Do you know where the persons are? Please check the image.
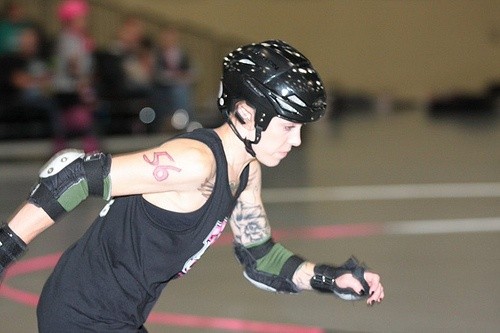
[0,0,193,157]
[0,39,385,333]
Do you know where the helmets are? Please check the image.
[218,39,327,124]
